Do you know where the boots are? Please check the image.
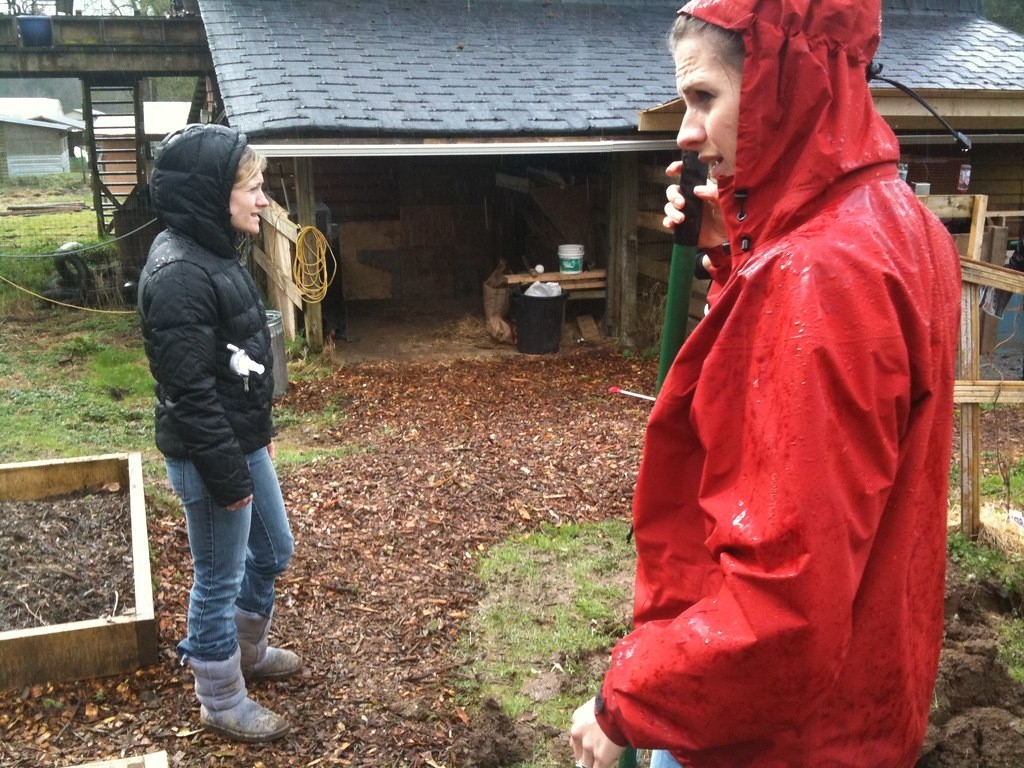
[188,641,289,741]
[234,606,303,679]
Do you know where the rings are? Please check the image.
[574,760,587,768]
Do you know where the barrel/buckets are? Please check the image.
[558,244,585,275]
[509,281,570,354]
[264,309,290,403]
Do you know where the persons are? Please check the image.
[694,248,713,280]
[135,123,302,739]
[566,0,962,768]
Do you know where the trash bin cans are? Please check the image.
[513,284,570,354]
[266,310,288,399]
